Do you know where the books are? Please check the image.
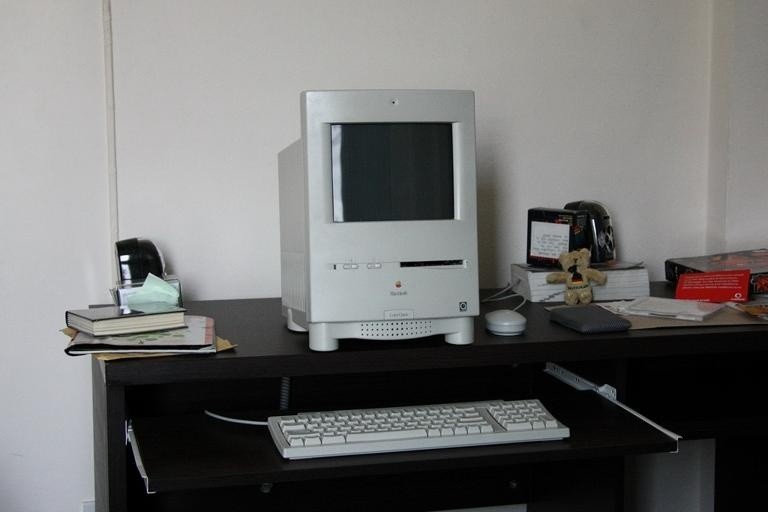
[618,295,725,324]
[508,257,651,304]
[720,293,768,321]
[63,314,218,357]
[64,303,188,338]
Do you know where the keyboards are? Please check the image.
[267,398,571,461]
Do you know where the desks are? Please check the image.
[91,297,768,511]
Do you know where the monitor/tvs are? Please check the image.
[277,88,480,352]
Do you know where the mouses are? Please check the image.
[485,309,527,336]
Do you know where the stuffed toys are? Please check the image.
[545,247,607,307]
